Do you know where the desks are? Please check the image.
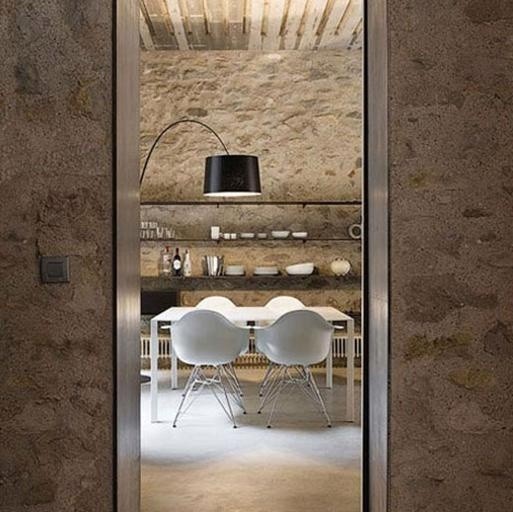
[148,306,356,424]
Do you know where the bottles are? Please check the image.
[162,246,191,277]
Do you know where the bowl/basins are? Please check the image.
[201,254,224,276]
[225,262,313,275]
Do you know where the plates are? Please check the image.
[239,231,308,240]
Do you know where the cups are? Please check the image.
[139,221,176,240]
[210,226,236,240]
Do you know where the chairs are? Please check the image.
[169,294,333,430]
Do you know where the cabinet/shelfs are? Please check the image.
[137,199,362,291]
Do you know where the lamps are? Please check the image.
[138,119,262,198]
